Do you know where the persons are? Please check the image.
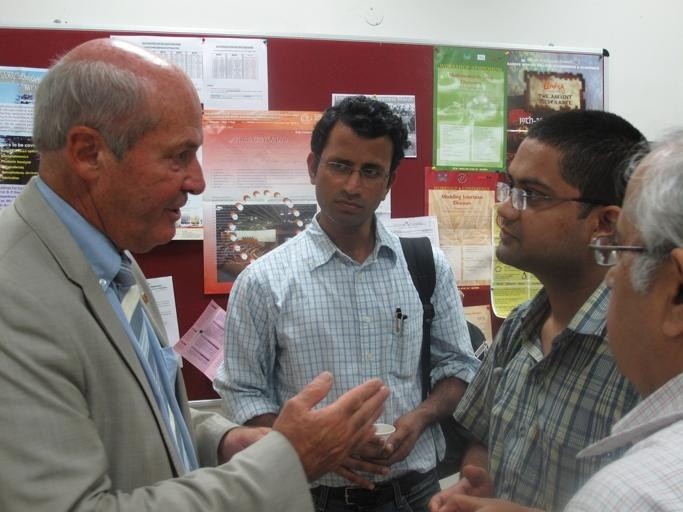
[0,38,395,512]
[422,109,652,512]
[561,127,682,512]
[209,93,482,511]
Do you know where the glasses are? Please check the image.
[591,238,649,266]
[495,181,607,212]
[314,153,393,187]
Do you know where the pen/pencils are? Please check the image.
[396,307,407,332]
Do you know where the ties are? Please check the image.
[111,255,193,474]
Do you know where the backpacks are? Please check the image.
[399,236,488,480]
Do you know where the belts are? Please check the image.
[311,466,435,508]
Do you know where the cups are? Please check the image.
[371,422,397,459]
